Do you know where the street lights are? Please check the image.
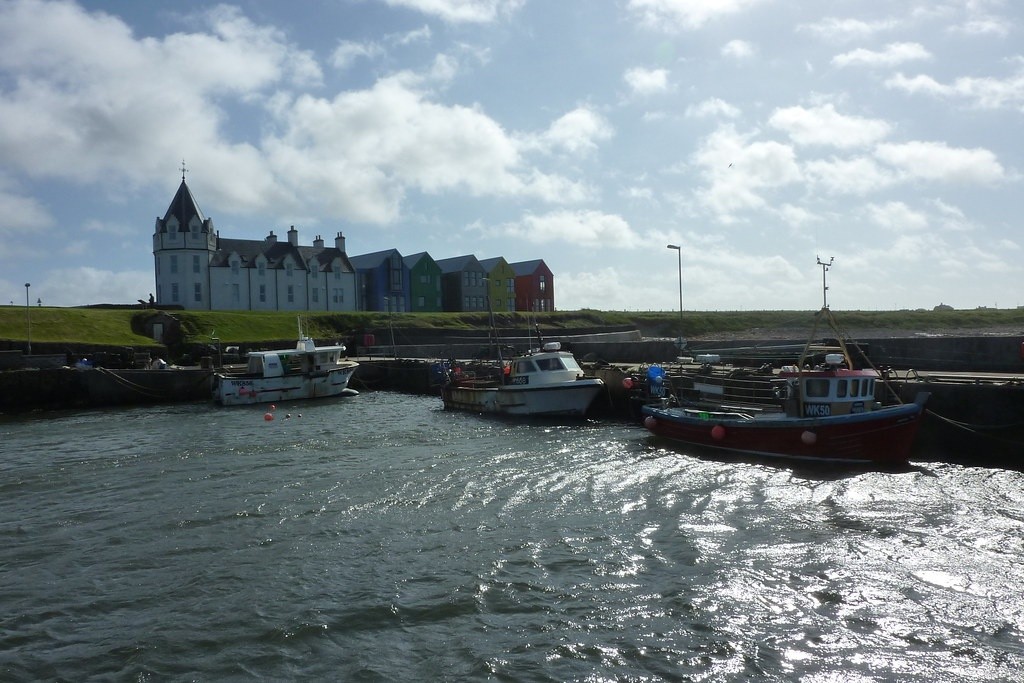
[25,282,32,354]
[667,245,682,358]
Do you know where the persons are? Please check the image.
[149,294,154,305]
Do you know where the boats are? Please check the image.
[212,314,360,408]
[430,277,607,425]
[622,254,922,464]
